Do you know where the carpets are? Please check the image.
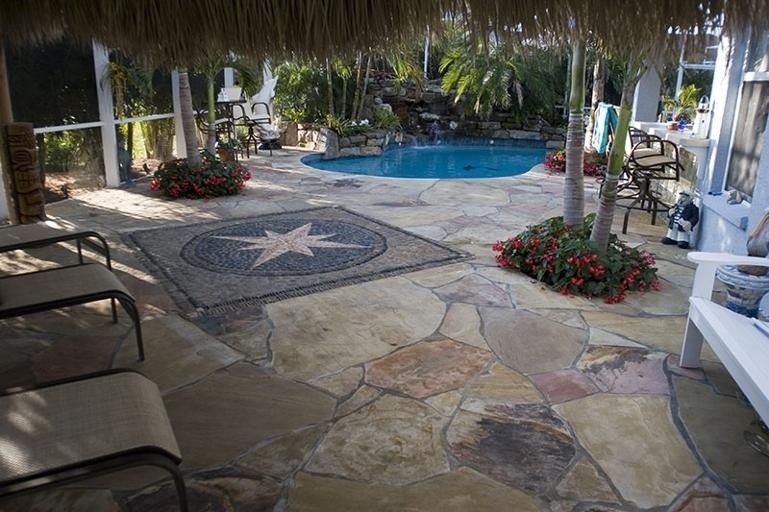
[118,203,476,320]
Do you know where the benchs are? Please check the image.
[676,252,769,431]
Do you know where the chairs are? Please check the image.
[0,219,112,271]
[192,99,275,160]
[598,101,685,236]
[1,367,191,512]
[1,262,145,362]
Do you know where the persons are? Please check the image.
[660,189,700,249]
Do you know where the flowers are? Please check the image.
[145,149,253,200]
[494,212,663,305]
[544,147,597,176]
